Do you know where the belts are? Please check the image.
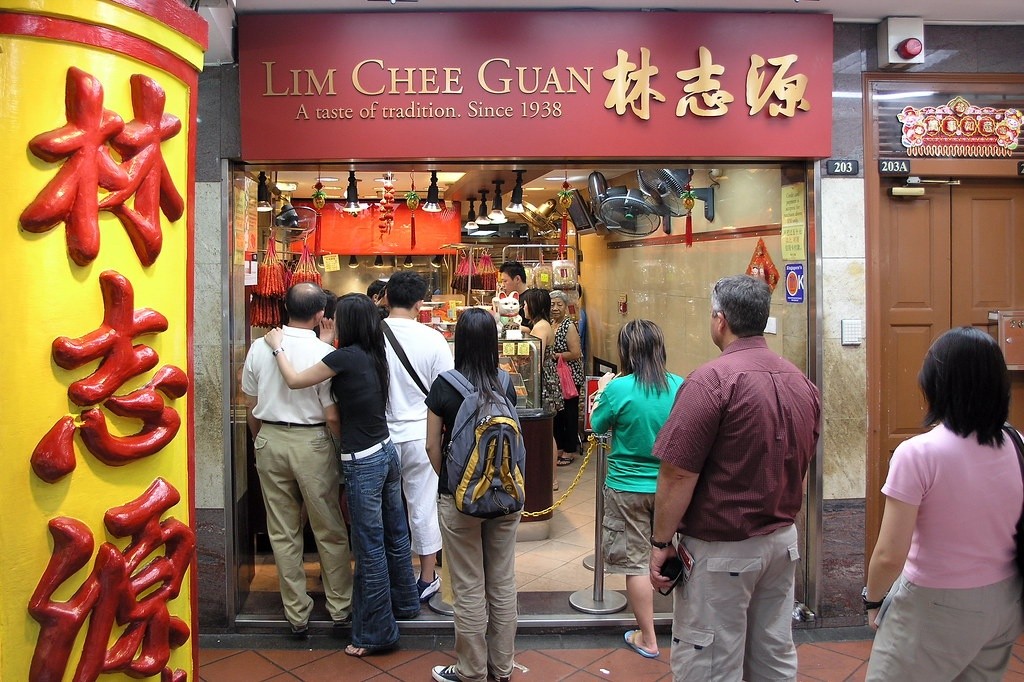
[262,419,326,427]
[340,436,391,462]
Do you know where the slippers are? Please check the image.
[557,457,574,466]
[624,629,660,658]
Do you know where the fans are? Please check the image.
[588,170,713,237]
[515,199,559,239]
[270,203,317,242]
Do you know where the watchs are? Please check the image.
[862,587,886,610]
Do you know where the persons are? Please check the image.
[263,293,420,655]
[425,308,522,682]
[590,319,684,658]
[498,261,534,331]
[649,274,820,682]
[382,271,453,601]
[523,289,566,491]
[368,281,390,318]
[241,284,354,635]
[315,290,337,338]
[861,327,1024,682]
[549,290,584,465]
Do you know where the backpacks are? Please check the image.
[438,369,527,519]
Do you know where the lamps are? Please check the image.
[319,255,442,269]
[257,170,445,212]
[465,169,524,229]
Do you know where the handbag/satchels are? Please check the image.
[556,353,580,400]
[1003,422,1024,577]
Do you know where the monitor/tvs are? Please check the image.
[565,188,596,236]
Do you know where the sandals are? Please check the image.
[345,644,375,656]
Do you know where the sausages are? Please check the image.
[251,265,323,329]
[450,270,498,293]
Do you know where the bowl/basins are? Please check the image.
[505,330,522,340]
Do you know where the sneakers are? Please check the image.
[415,568,442,604]
[489,667,510,682]
[432,665,463,682]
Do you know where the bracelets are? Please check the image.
[594,391,602,403]
[272,347,284,356]
[650,536,672,548]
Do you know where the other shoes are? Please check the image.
[332,613,352,627]
[289,621,308,635]
